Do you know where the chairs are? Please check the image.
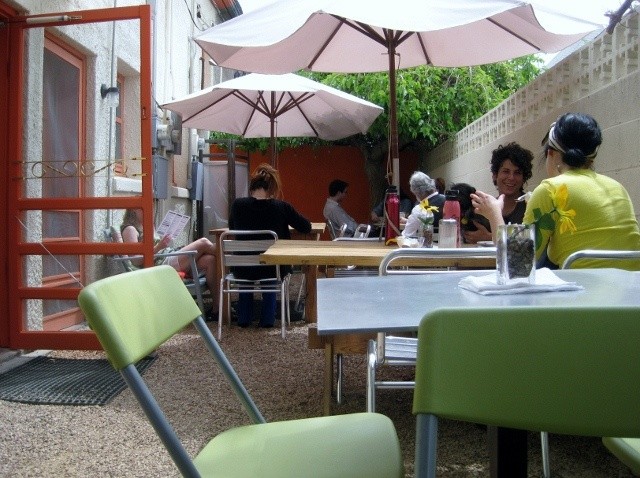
[325,219,337,239]
[72,266,402,477]
[411,306,639,478]
[365,247,501,415]
[215,230,294,341]
[106,227,213,323]
[293,224,370,321]
[332,223,347,241]
[556,251,640,272]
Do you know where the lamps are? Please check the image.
[101,84,117,99]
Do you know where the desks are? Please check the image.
[313,269,639,477]
[209,223,327,325]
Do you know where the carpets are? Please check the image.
[1,357,155,407]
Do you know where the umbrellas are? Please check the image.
[159,73,385,171]
[191,0,607,197]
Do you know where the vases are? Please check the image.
[421,225,435,247]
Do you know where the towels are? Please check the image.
[458,267,583,296]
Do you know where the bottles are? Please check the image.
[438,219,457,248]
[385,185,400,245]
[443,190,461,247]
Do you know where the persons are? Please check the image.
[370,189,412,222]
[463,143,533,243]
[323,179,359,238]
[402,171,445,243]
[120,208,217,314]
[228,164,311,329]
[469,113,640,272]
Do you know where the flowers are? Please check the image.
[417,199,439,229]
[512,183,577,249]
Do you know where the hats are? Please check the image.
[541,123,599,158]
